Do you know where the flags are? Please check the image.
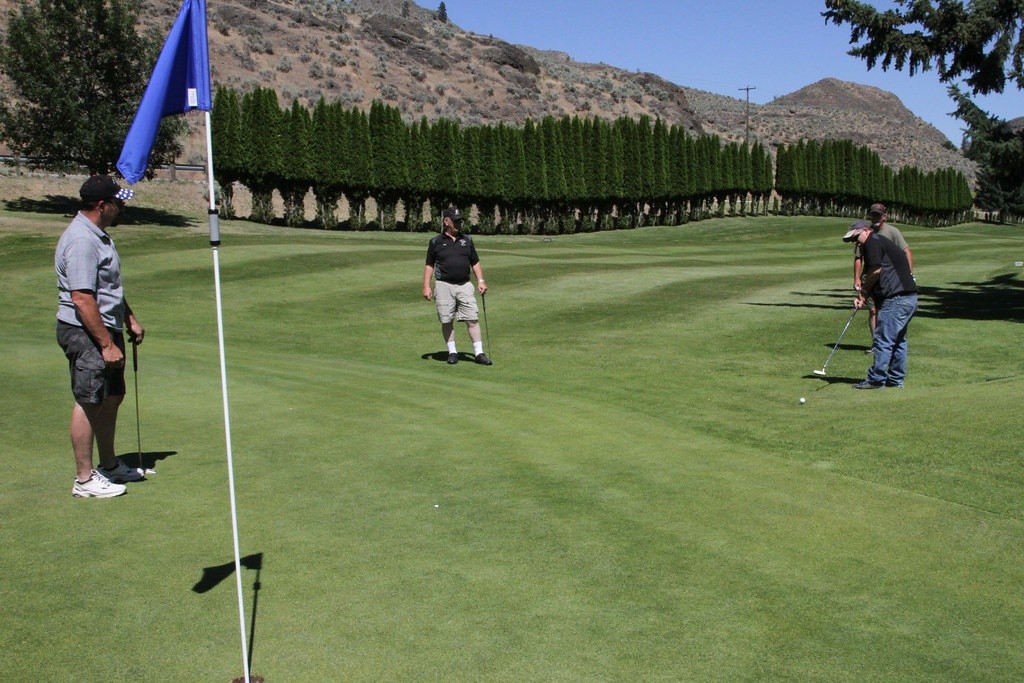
[117,0,214,184]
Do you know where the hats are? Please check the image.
[842,220,868,243]
[870,204,886,214]
[444,207,466,221]
[80,174,134,202]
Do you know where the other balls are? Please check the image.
[800,398,805,403]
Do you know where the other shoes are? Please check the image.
[865,347,876,353]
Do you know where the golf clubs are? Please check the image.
[482,293,491,360]
[813,305,860,376]
[132,338,157,474]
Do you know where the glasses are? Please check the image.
[448,217,463,223]
[104,200,126,208]
[850,234,859,241]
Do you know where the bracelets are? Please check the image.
[478,279,486,283]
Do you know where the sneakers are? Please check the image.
[884,382,904,389]
[71,468,127,499]
[475,353,492,365]
[853,381,883,389]
[447,353,457,364]
[95,456,144,482]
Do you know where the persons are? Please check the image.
[422,207,493,366]
[54,174,145,497]
[854,204,912,354]
[843,222,918,389]
